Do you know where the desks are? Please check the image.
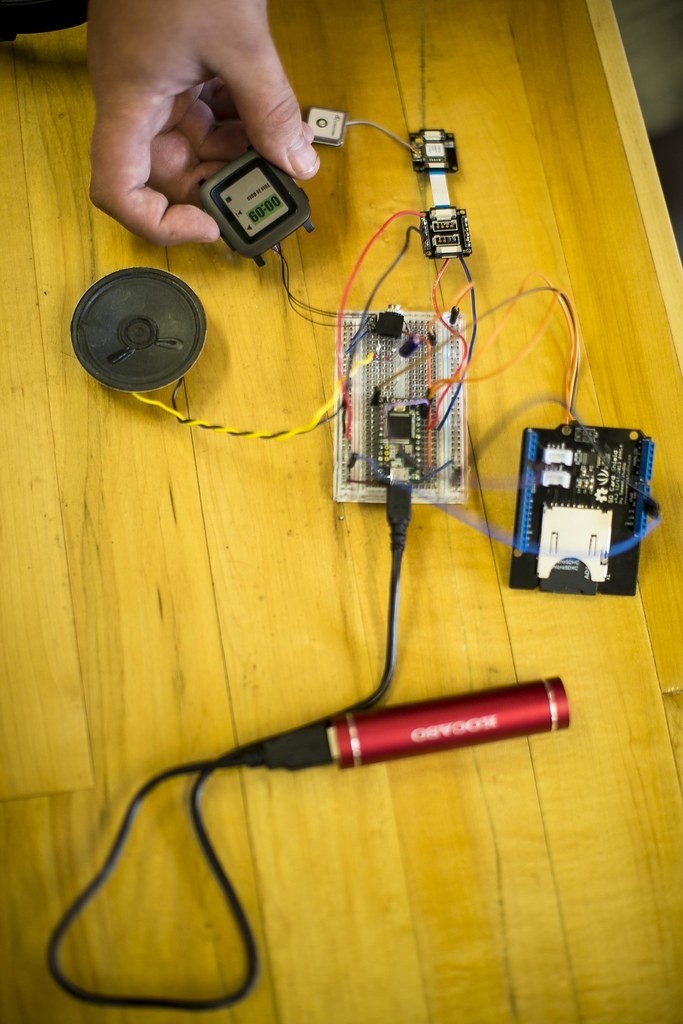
[0,3,682,1024]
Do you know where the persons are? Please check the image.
[0,0,320,247]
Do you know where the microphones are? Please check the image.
[238,683,570,774]
